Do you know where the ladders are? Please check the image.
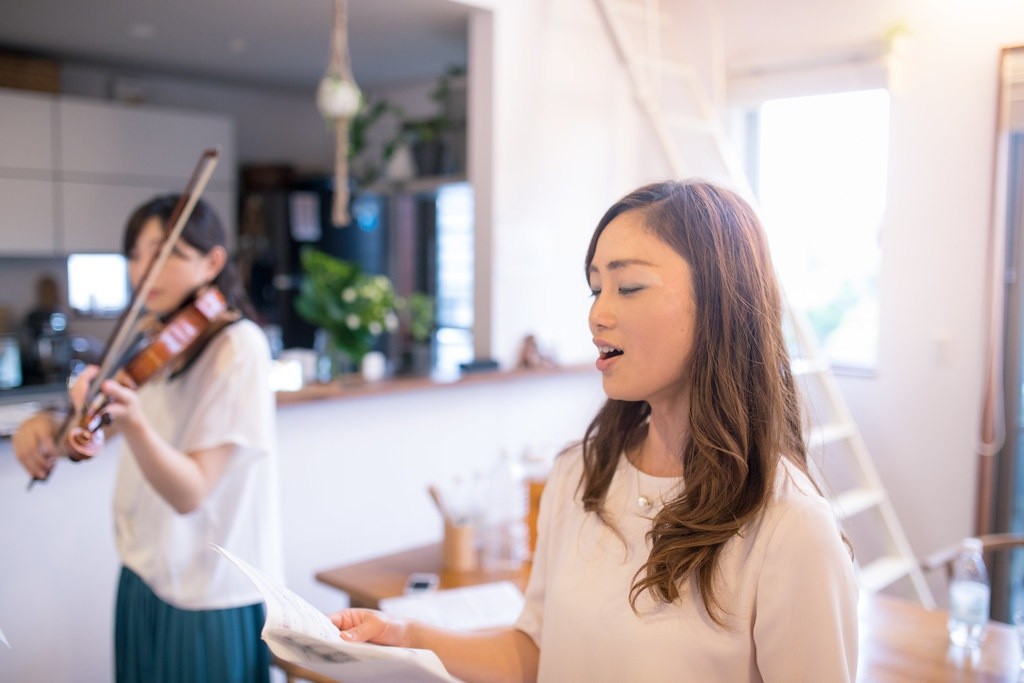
[593,0,935,613]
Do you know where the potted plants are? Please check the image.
[299,250,433,392]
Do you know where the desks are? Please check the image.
[315,517,1024,683]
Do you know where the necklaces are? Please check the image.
[636,429,685,511]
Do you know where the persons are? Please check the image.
[323,174,866,683]
[21,276,97,386]
[9,195,287,683]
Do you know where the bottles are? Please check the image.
[948,538,991,649]
[482,449,530,569]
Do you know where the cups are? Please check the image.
[444,519,475,571]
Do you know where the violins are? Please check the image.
[61,284,229,463]
